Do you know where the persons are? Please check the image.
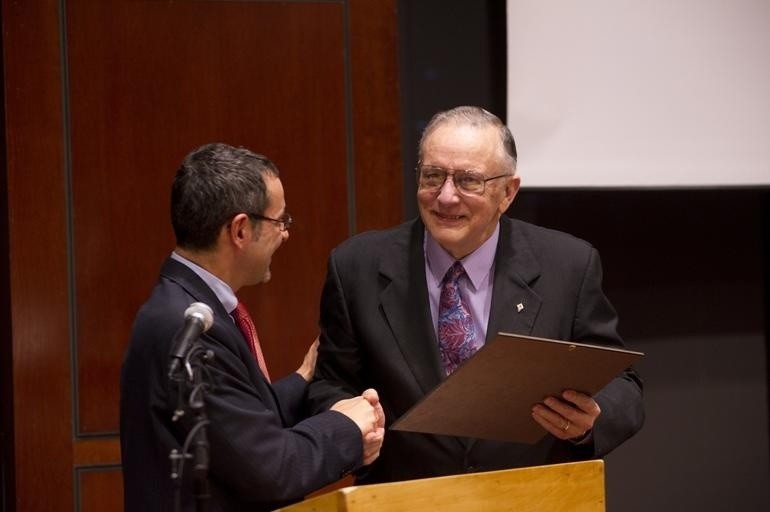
[301,106,645,483]
[120,141,379,512]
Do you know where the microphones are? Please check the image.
[165,301,214,380]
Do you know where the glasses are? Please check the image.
[415,163,514,199]
[226,210,292,232]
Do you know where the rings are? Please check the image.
[563,420,570,433]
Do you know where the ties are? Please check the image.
[229,302,272,387]
[437,263,478,377]
